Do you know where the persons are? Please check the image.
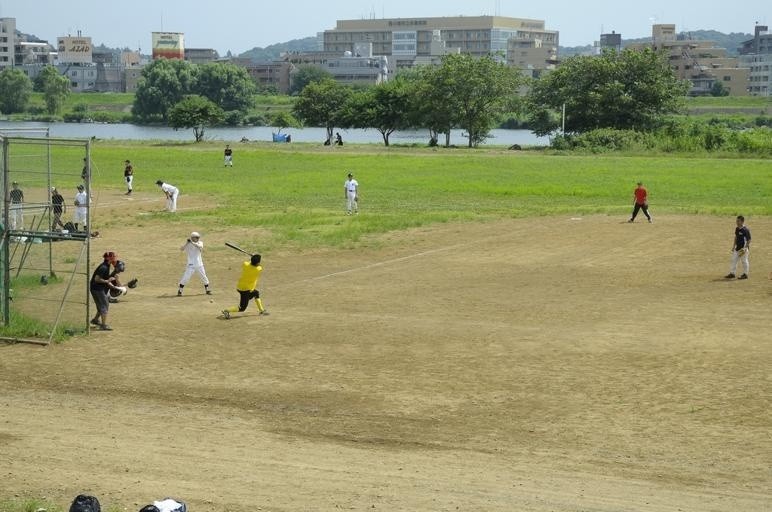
[80,158,92,188]
[331,131,344,146]
[177,231,213,296]
[224,143,234,167]
[73,185,89,229]
[90,252,127,332]
[156,180,179,210]
[345,171,359,215]
[629,182,655,223]
[123,159,133,195]
[222,254,270,320]
[726,214,752,283]
[48,185,68,227]
[69,494,101,512]
[8,181,26,227]
[139,504,160,512]
[107,262,137,304]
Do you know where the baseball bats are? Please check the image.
[225,243,253,255]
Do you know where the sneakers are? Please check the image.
[725,272,751,282]
[345,210,359,217]
[205,290,213,296]
[161,207,177,213]
[628,217,655,225]
[257,309,271,317]
[124,192,133,196]
[90,317,113,332]
[220,308,230,321]
[177,289,184,297]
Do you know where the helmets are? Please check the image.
[101,251,118,262]
[114,259,125,273]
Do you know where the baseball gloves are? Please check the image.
[128,279,138,288]
[737,247,748,258]
[229,157,232,161]
[127,177,130,182]
[354,195,359,202]
[642,201,648,209]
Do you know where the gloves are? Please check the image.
[126,177,131,183]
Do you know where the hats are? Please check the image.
[155,179,164,185]
[124,159,131,164]
[11,180,20,186]
[50,186,56,194]
[190,231,200,239]
[347,172,353,177]
[76,184,85,192]
